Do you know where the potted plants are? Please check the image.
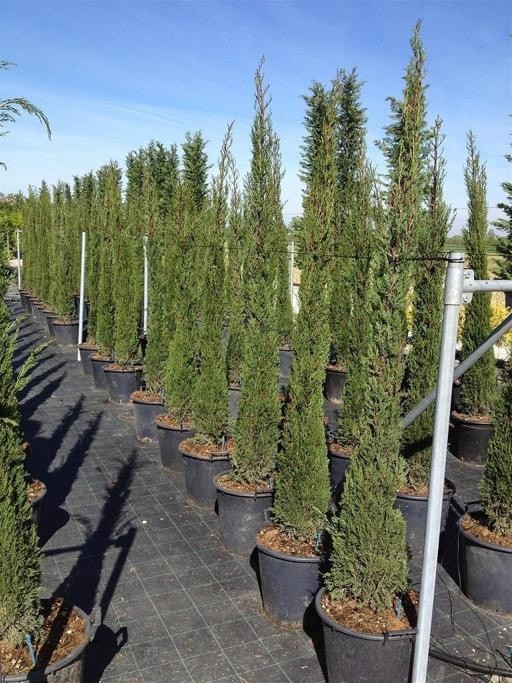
[0,18,512,683]
